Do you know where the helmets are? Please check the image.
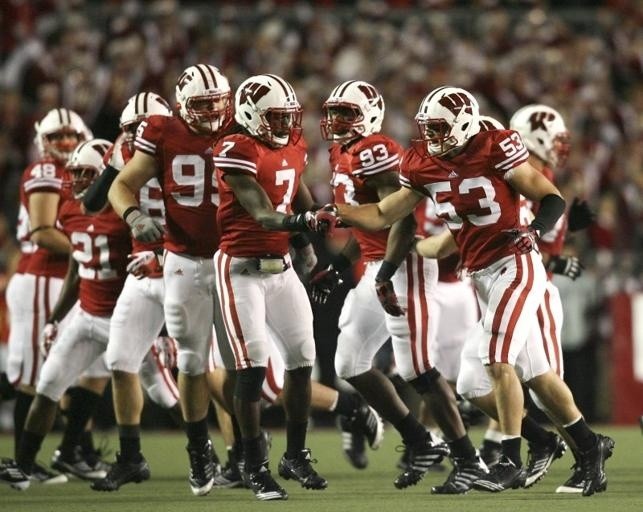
[69,135,116,196]
[412,85,480,157]
[510,103,569,168]
[320,80,386,146]
[36,107,93,162]
[174,63,232,132]
[119,92,174,156]
[481,113,507,131]
[234,72,304,147]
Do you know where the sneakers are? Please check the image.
[471,454,523,490]
[243,462,288,501]
[555,458,585,493]
[479,441,500,462]
[50,443,107,481]
[522,431,567,488]
[278,448,328,489]
[0,456,31,491]
[19,461,68,484]
[186,439,214,497]
[350,394,384,451]
[215,452,246,488]
[580,432,615,497]
[430,456,490,494]
[90,452,151,492]
[393,432,450,489]
[336,413,368,469]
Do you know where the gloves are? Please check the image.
[309,252,352,304]
[126,250,162,280]
[314,202,340,239]
[121,206,166,244]
[568,197,594,231]
[282,209,315,235]
[151,326,176,370]
[40,317,58,361]
[545,253,584,281]
[501,222,544,256]
[374,260,406,318]
